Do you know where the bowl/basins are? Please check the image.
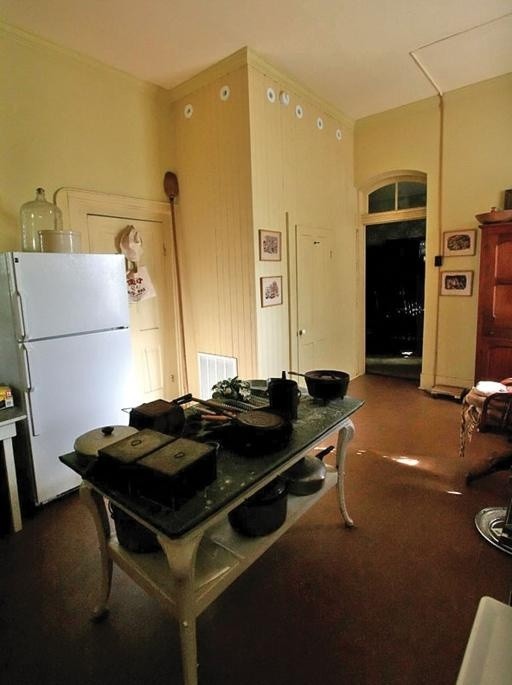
[304,370,350,405]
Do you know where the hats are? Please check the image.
[119,226,143,262]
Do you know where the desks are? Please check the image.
[56,383,367,685]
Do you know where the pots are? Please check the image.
[227,476,288,538]
[37,230,81,254]
[198,406,293,449]
[276,445,335,497]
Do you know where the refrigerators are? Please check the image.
[0,250,143,507]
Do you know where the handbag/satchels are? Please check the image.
[466,380,507,407]
[127,265,156,303]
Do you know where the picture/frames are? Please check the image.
[258,228,283,309]
[438,227,477,297]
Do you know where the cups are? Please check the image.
[268,380,299,420]
